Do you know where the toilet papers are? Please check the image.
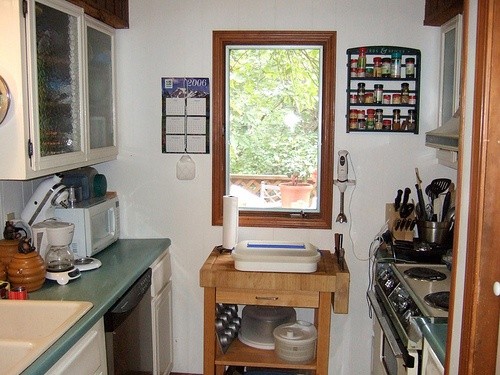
[222,194,239,250]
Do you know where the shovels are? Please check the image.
[430,178,451,199]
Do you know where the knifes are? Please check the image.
[393,188,417,233]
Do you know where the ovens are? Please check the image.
[367,264,426,375]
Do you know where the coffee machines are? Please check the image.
[32,221,82,286]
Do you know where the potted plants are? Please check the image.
[279,158,314,209]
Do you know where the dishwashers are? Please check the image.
[98,267,157,374]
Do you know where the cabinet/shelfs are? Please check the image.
[436,13,464,172]
[345,45,422,135]
[0,0,119,182]
[113,246,174,375]
[198,244,352,375]
[43,315,109,375]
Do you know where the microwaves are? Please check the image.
[43,190,122,262]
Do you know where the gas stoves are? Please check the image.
[388,262,453,322]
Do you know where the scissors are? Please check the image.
[399,203,414,218]
